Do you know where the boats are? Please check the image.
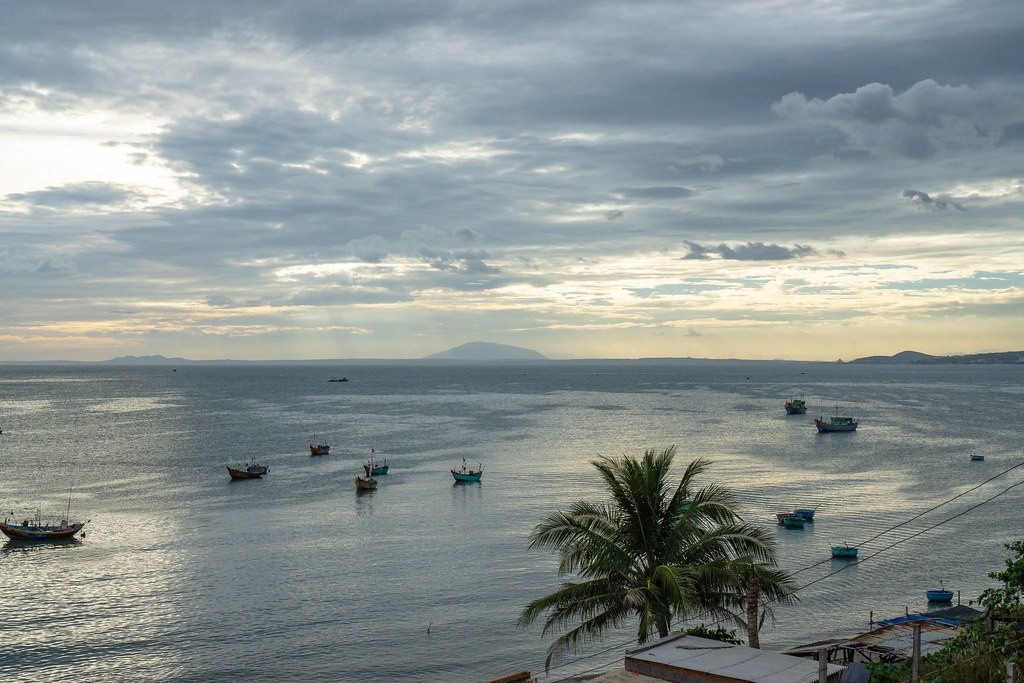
[225,465,261,479]
[794,509,814,519]
[831,546,858,557]
[308,445,330,455]
[355,476,378,489]
[969,453,986,462]
[813,418,858,432]
[363,463,389,476]
[775,512,794,522]
[247,465,270,474]
[784,517,805,526]
[925,589,954,601]
[327,377,350,384]
[450,469,483,481]
[782,399,808,414]
[0,510,97,540]
